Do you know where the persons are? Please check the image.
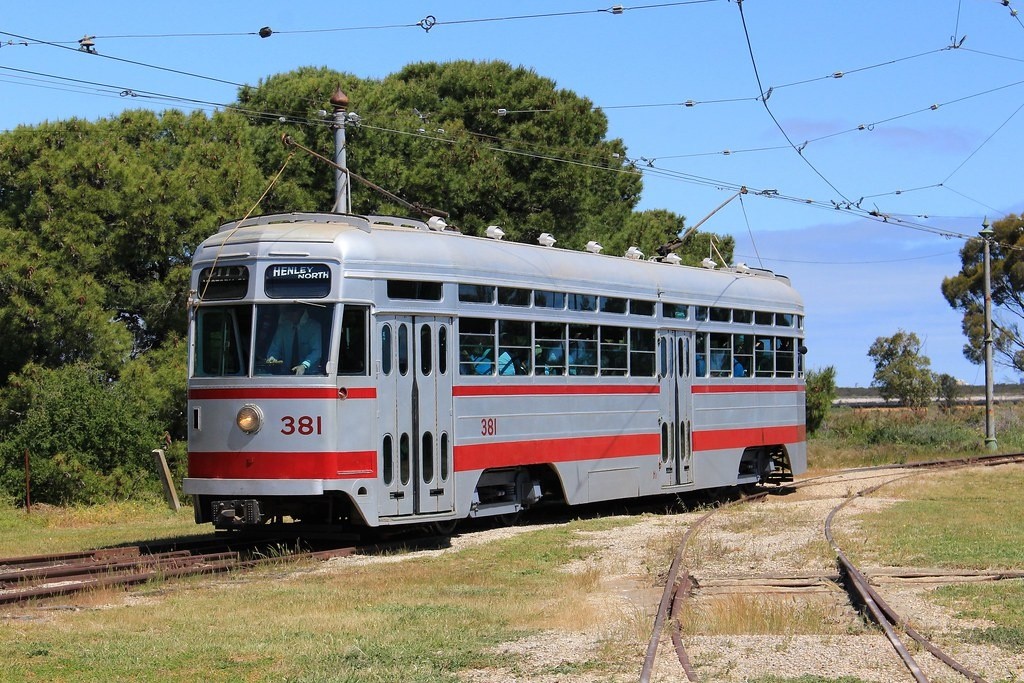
[265,304,323,375]
[460,327,746,377]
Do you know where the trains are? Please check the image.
[153,129,808,544]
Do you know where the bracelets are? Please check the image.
[302,364,308,370]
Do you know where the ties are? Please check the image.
[291,325,300,370]
